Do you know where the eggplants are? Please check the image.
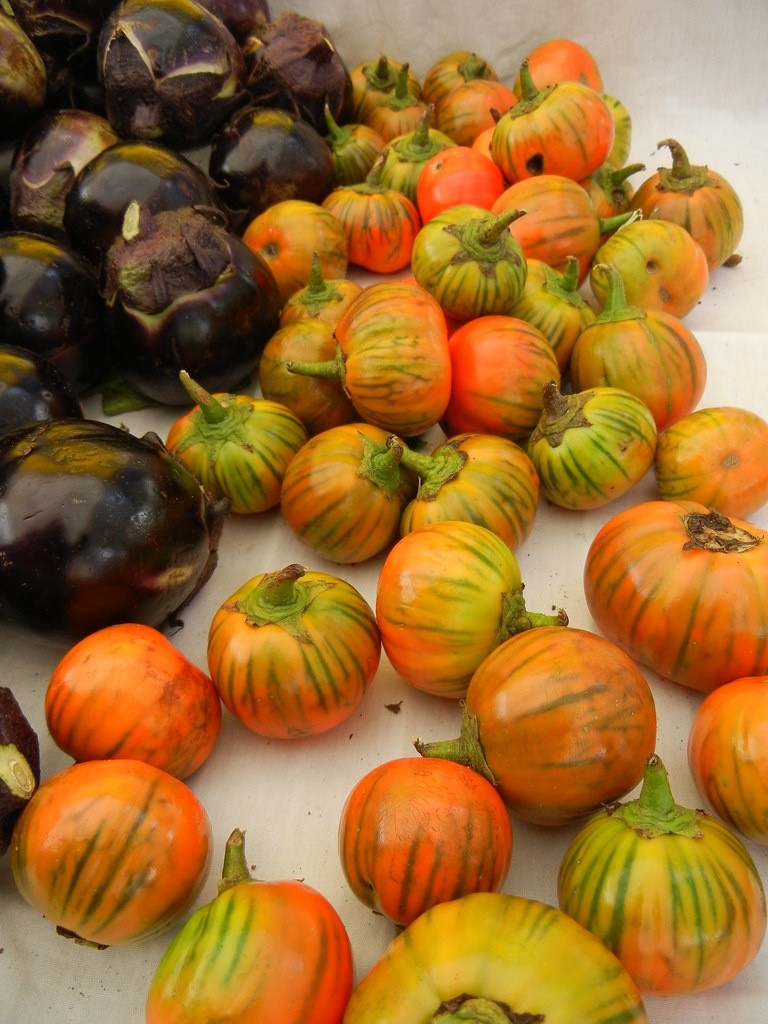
[0,0,357,828]
